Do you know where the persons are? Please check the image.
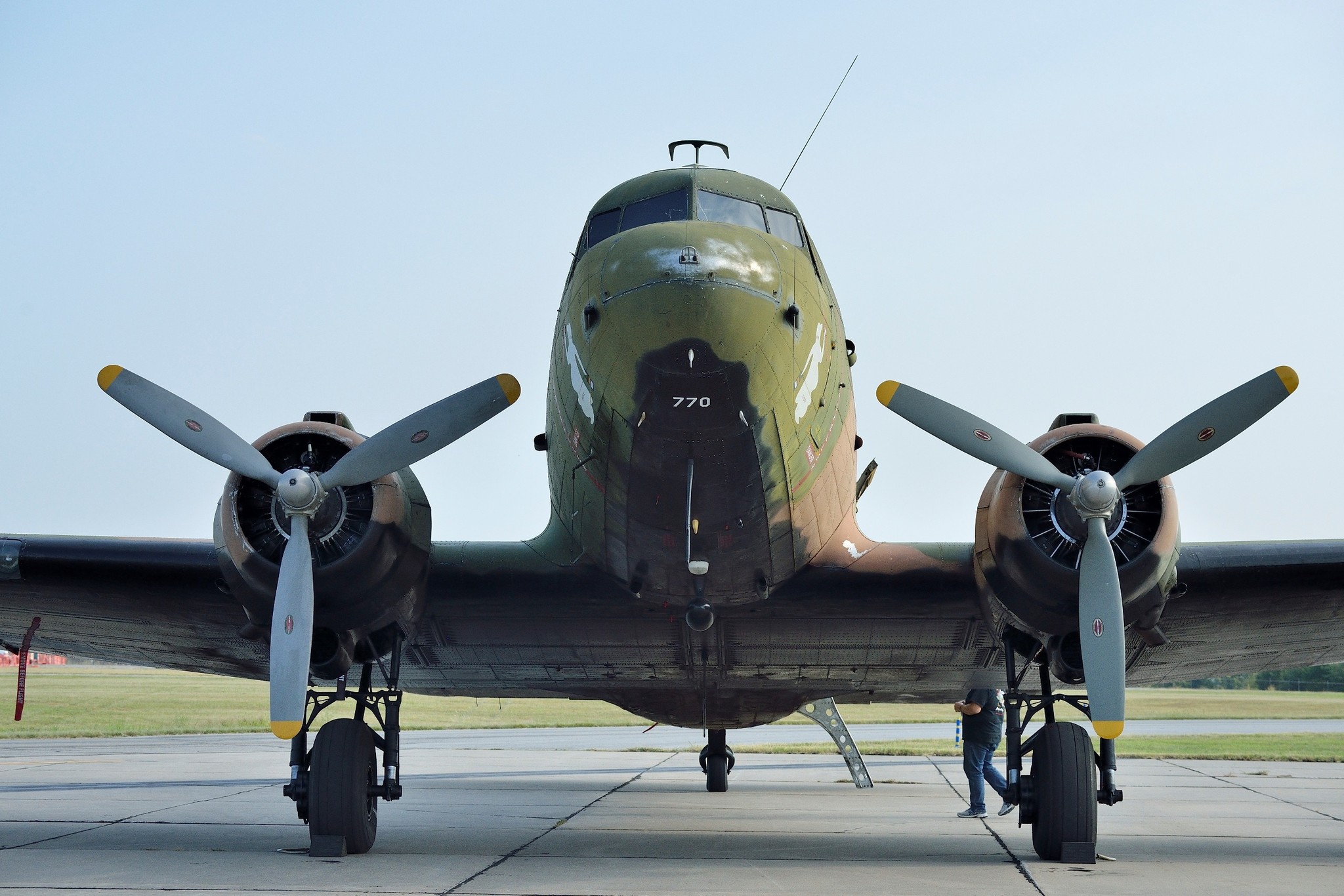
[953,688,1015,818]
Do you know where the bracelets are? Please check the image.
[960,704,964,711]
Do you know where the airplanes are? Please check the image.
[0,135,1343,866]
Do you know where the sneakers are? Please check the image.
[998,801,1015,816]
[957,808,988,818]
